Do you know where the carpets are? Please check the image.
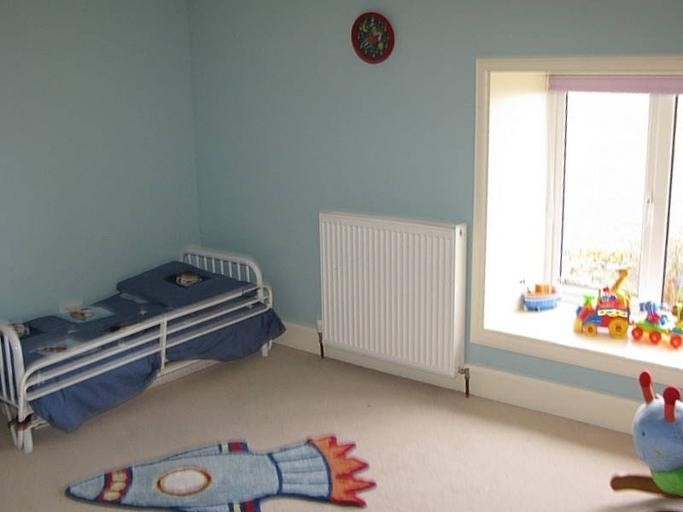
[63,433,376,512]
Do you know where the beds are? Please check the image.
[1,248,275,456]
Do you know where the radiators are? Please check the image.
[317,211,468,379]
